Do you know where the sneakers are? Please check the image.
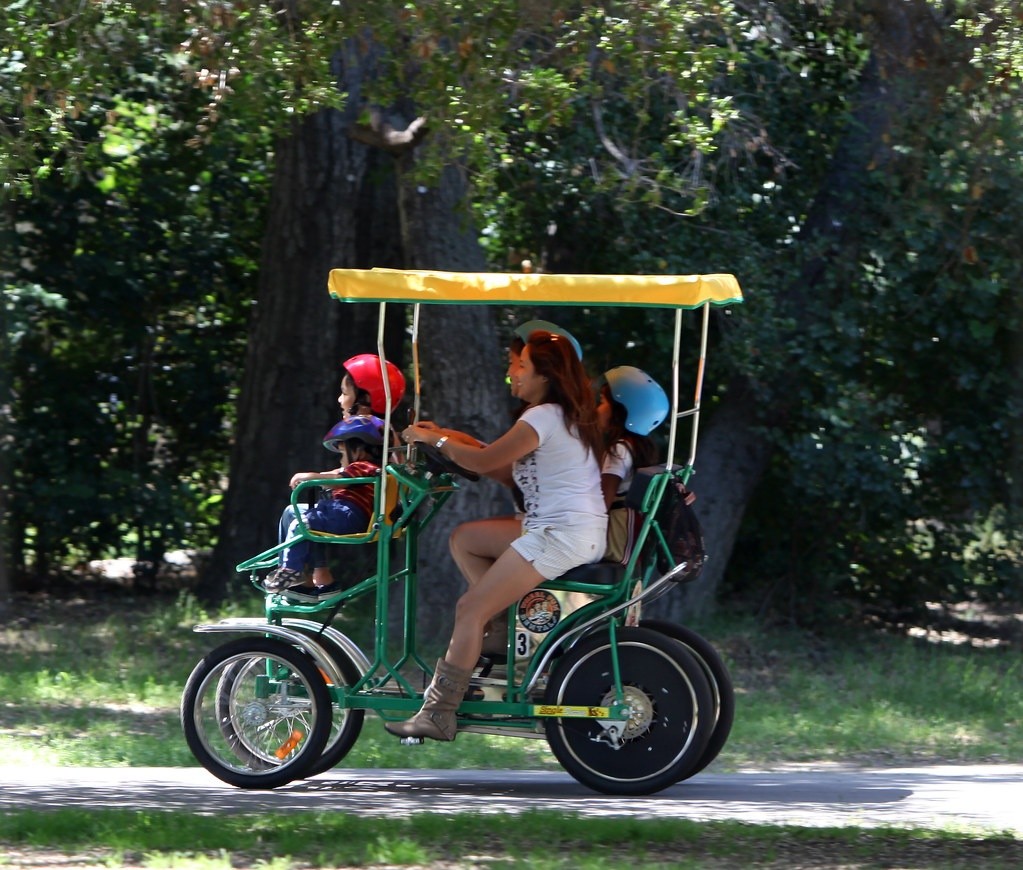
[261,568,308,592]
[278,580,343,603]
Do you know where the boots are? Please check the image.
[442,608,509,665]
[384,657,472,741]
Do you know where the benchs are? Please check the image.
[290,463,463,545]
[505,463,695,705]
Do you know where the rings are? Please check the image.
[405,436,409,439]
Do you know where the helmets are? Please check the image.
[513,320,582,362]
[343,354,406,414]
[604,366,669,436]
[322,414,394,454]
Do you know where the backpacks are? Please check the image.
[657,478,705,582]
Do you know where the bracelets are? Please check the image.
[435,436,448,449]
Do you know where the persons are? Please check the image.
[505,319,670,567]
[382,327,623,743]
[265,415,394,593]
[277,352,407,603]
